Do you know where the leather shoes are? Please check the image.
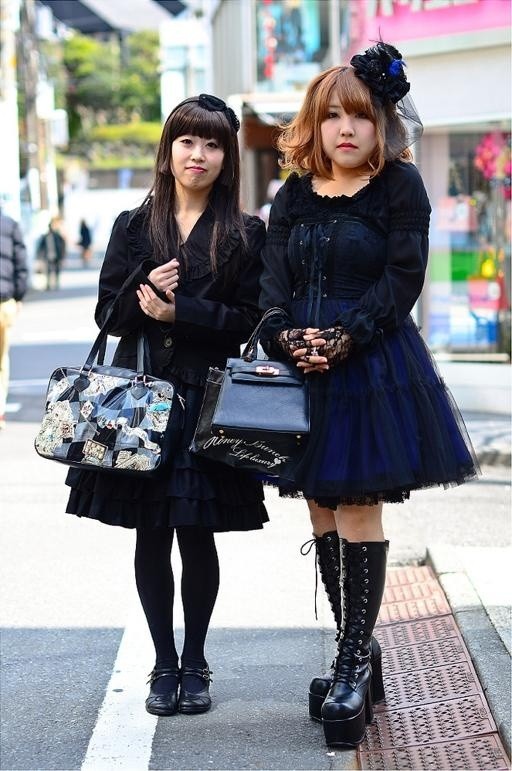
[145,668,180,715]
[178,662,211,712]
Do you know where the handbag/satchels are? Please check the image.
[34,362,187,471]
[190,357,323,483]
[212,357,313,443]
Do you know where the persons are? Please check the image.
[37,222,65,292]
[257,40,480,751]
[65,95,266,712]
[0,204,30,430]
[77,219,91,270]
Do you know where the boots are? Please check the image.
[309,530,384,721]
[320,538,389,746]
